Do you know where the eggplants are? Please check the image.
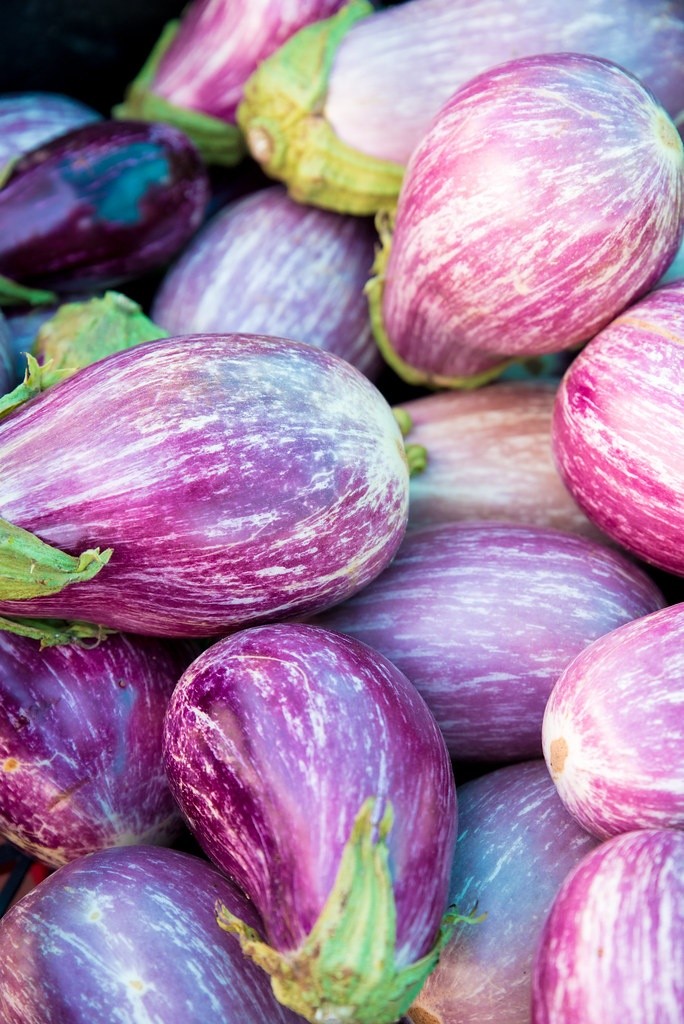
[1,0,684,1024]
